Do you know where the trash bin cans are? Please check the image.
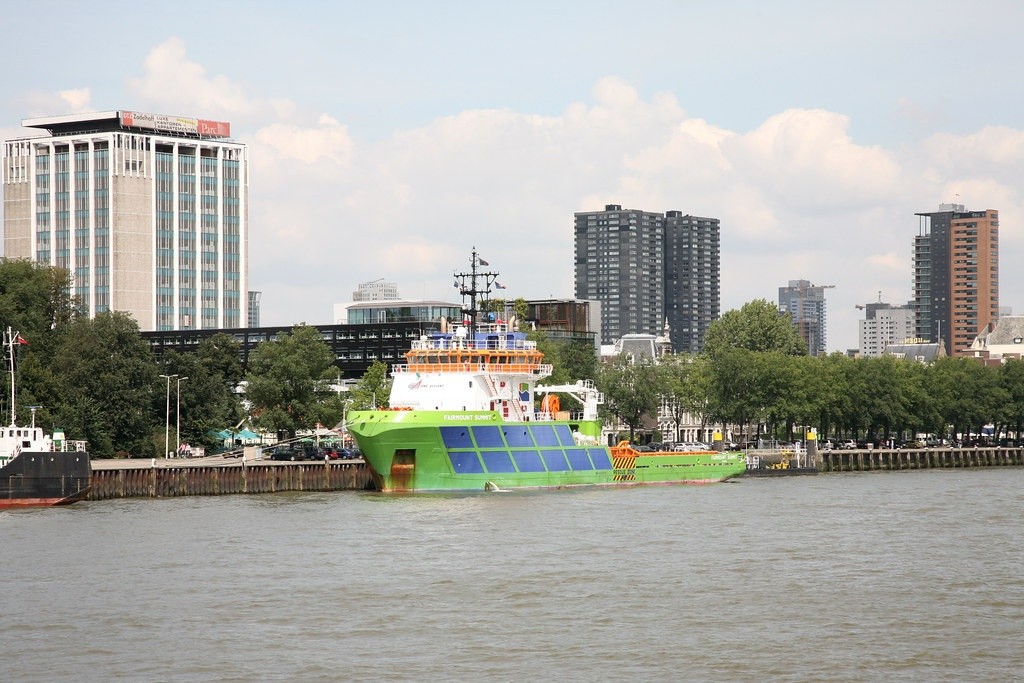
[169,450,174,459]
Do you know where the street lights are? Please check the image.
[176,377,188,459]
[158,373,178,460]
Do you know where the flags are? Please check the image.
[453,281,458,287]
[480,259,489,265]
[17,335,31,346]
[495,282,505,289]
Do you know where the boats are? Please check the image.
[342,244,748,494]
[0,323,93,510]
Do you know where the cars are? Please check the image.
[270,441,364,461]
[629,435,782,453]
[819,431,953,449]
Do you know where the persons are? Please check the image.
[879,436,891,449]
[654,444,674,451]
[958,439,963,448]
[333,443,337,449]
[843,441,847,449]
[932,435,936,440]
[179,442,191,458]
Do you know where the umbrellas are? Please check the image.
[298,437,343,445]
[217,428,259,447]
